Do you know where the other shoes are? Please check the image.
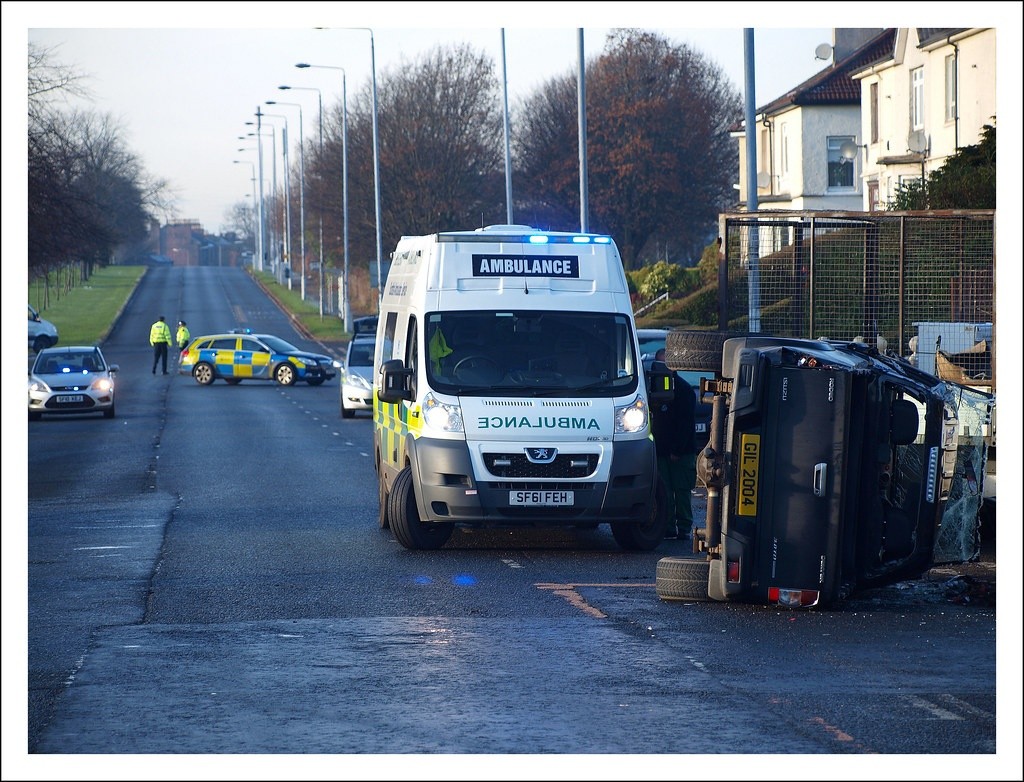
[664,531,677,537]
[163,371,169,375]
[677,530,690,540]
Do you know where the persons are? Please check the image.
[150,316,172,375]
[177,321,190,353]
[656,348,697,540]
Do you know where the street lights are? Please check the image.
[233,112,293,288]
[294,62,351,332]
[278,85,327,319]
[266,100,306,302]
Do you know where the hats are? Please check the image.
[178,321,186,325]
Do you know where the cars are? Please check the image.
[635,327,715,446]
[28,345,120,419]
[338,315,395,418]
[176,326,337,388]
[28,304,59,353]
[652,327,995,613]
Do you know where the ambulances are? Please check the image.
[370,223,675,554]
